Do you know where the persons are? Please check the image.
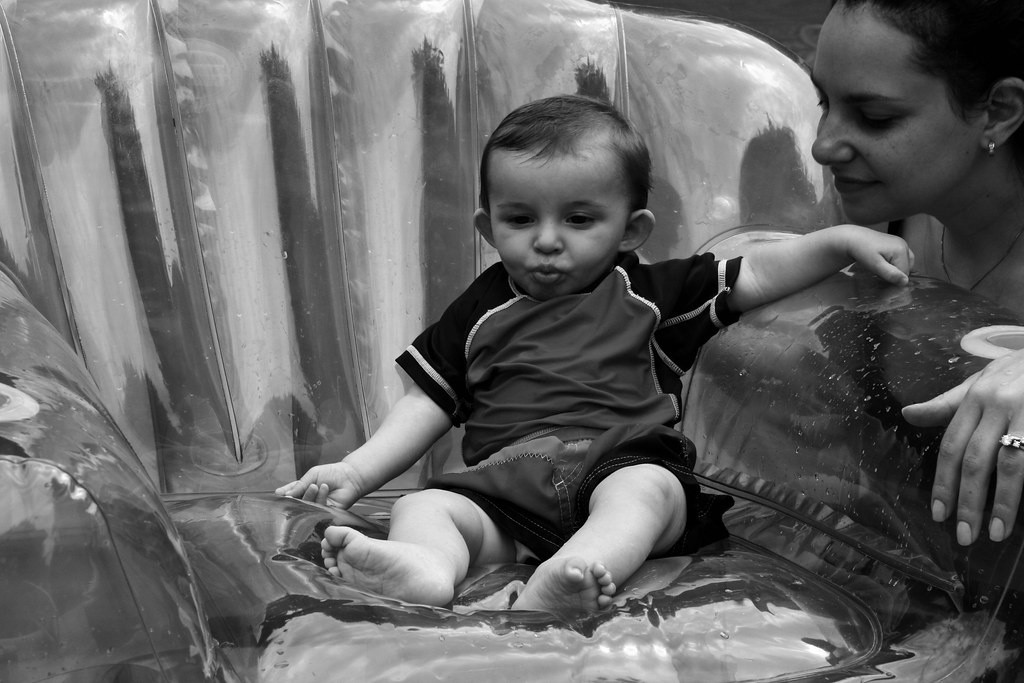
[810,1,1024,545]
[276,95,915,612]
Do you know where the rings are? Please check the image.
[999,434,1024,452]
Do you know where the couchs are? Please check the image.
[0,0,1024,683]
